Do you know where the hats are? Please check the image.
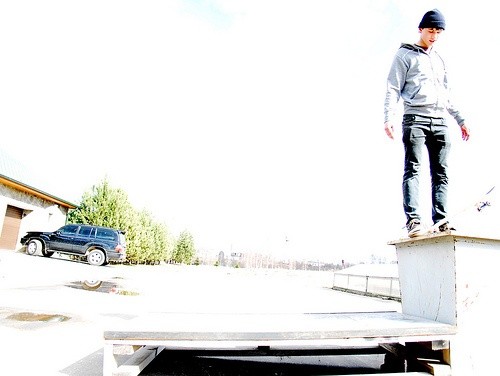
[418,8,446,30]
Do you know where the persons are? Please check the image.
[384,8,471,238]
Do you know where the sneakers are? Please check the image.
[402,217,427,237]
[431,219,458,230]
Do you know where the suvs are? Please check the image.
[20,223,128,267]
